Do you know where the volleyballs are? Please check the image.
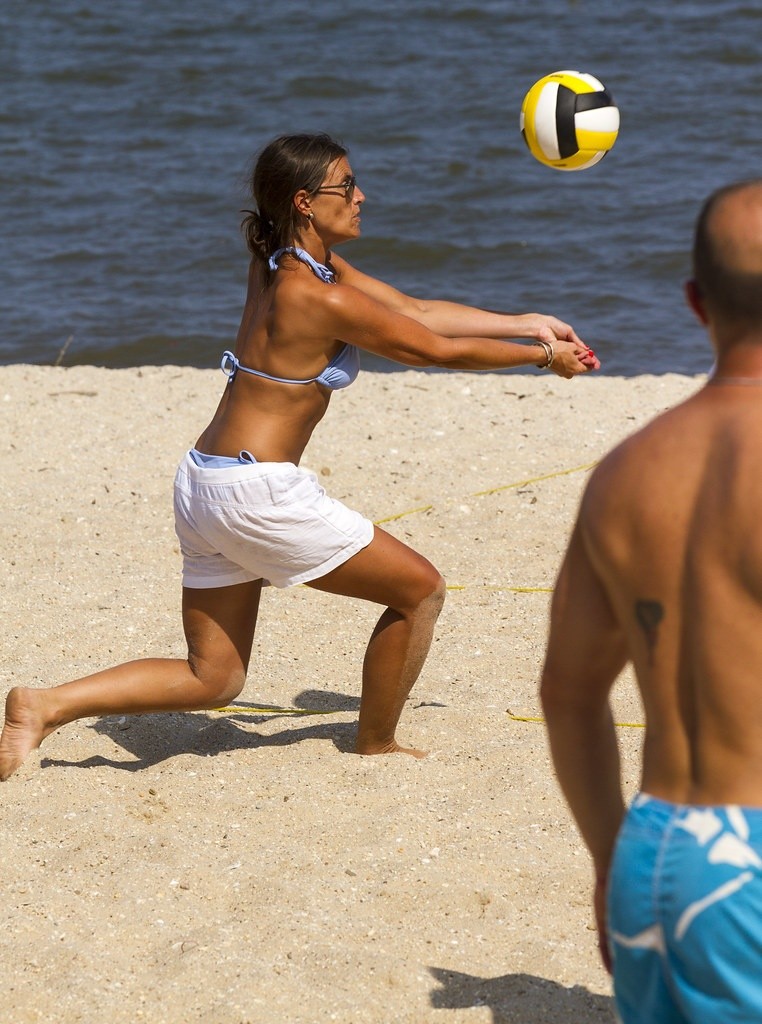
[519,69,621,173]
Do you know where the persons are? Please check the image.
[539,180,762,1024]
[0,134,601,780]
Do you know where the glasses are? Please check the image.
[317,176,355,202]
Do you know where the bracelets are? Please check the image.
[532,341,554,371]
